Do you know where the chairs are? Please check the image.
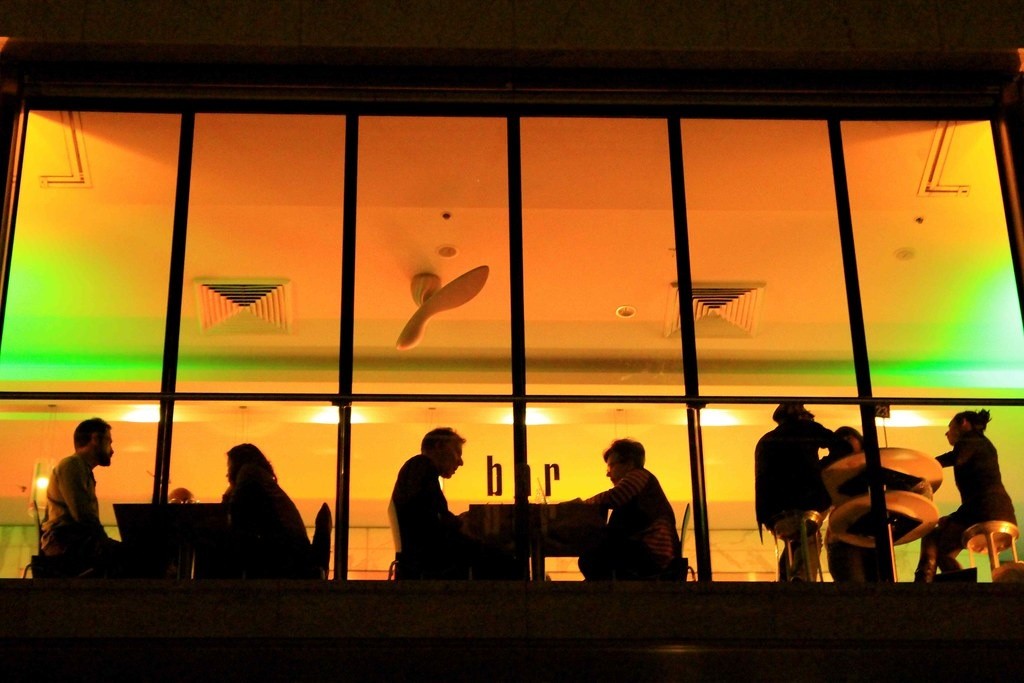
[313,503,334,586]
[25,498,117,580]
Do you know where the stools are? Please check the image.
[773,512,828,583]
[964,519,1020,584]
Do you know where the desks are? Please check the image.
[115,503,234,594]
[470,503,607,580]
[823,447,944,578]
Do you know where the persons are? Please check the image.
[392,428,517,581]
[40,419,139,579]
[755,402,1016,583]
[221,444,319,579]
[578,440,681,581]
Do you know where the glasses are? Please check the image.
[607,464,617,474]
